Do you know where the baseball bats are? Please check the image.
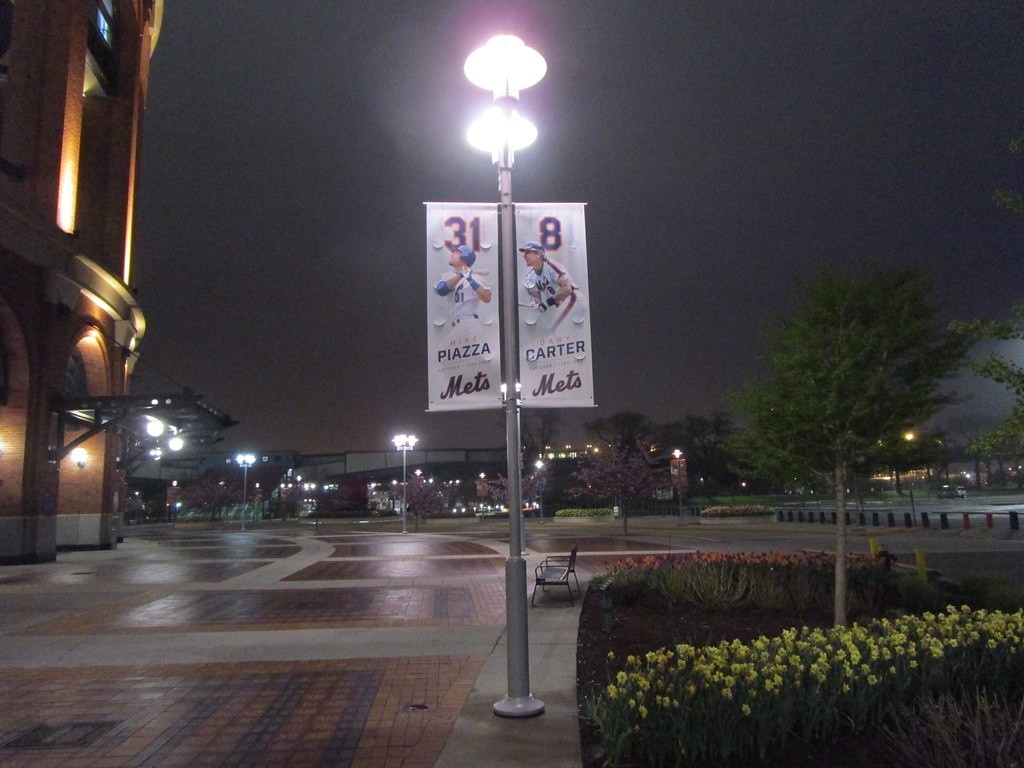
[473,269,490,276]
[518,302,534,308]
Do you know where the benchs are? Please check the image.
[530,544,579,608]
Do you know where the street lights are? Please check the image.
[461,32,550,722]
[235,455,256,533]
[534,460,546,525]
[391,434,419,535]
[671,449,688,526]
[280,483,293,521]
[171,480,178,529]
[478,472,486,522]
[296,476,302,527]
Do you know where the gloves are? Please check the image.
[535,301,550,313]
[458,268,475,281]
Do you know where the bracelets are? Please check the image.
[471,283,480,290]
[546,297,555,306]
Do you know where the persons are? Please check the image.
[518,242,587,338]
[436,245,490,337]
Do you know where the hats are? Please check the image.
[518,242,545,255]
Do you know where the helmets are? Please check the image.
[451,245,476,266]
